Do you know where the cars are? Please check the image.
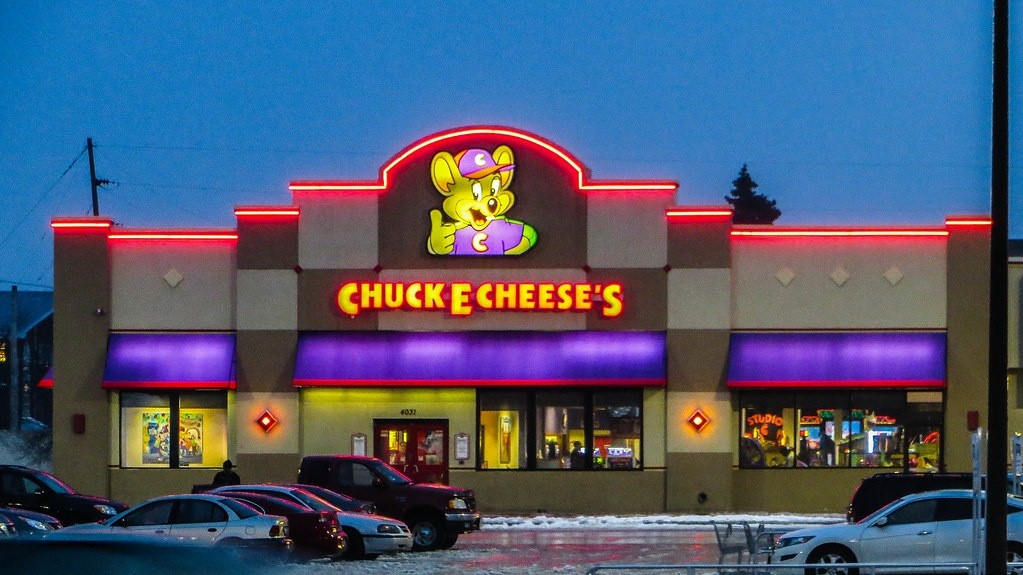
[768,488,1023,575]
[0,507,63,537]
[264,482,378,516]
[49,493,292,575]
[202,485,414,562]
[205,491,346,561]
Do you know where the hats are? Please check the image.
[223,460,236,468]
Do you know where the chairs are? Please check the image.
[712,520,788,574]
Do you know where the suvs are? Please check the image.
[0,464,133,528]
[846,470,1023,524]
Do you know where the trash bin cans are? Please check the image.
[191,483,210,494]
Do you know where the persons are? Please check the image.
[213,460,240,490]
[818,432,828,462]
[571,441,584,468]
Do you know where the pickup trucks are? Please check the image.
[190,453,481,552]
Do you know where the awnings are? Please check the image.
[293,333,669,388]
[38,368,53,387]
[726,331,948,388]
[101,332,237,389]
